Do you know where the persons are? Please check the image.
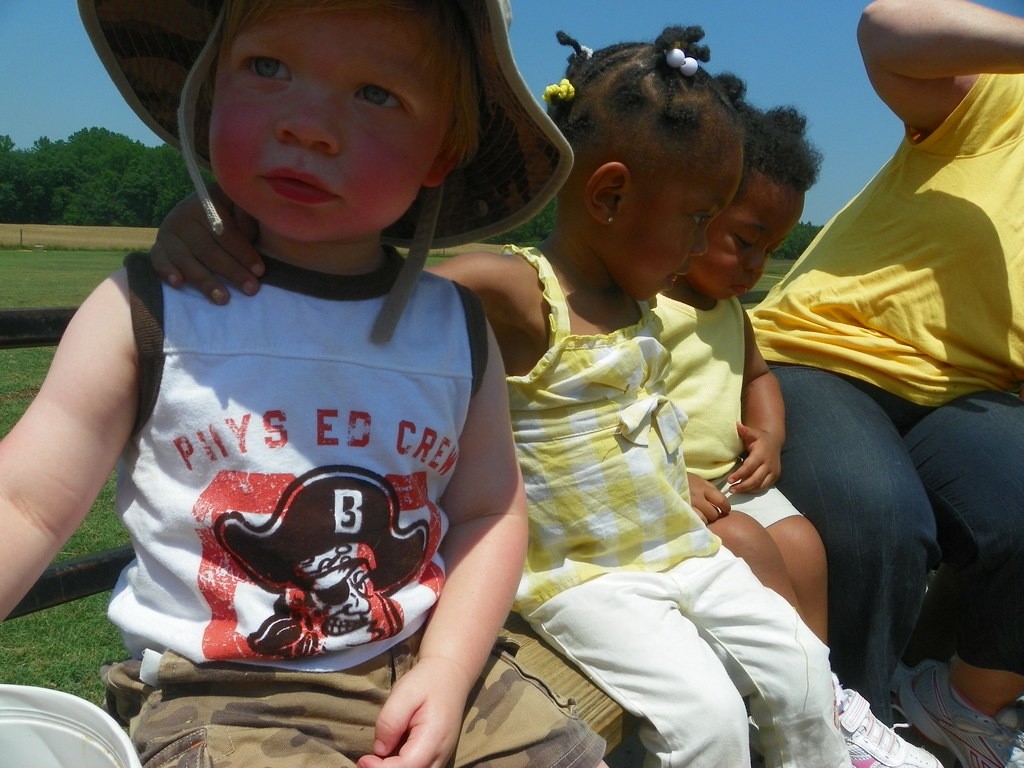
[0,0,608,767]
[740,0,1024,768]
[649,101,945,768]
[151,24,853,768]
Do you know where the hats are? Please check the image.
[77,0,574,345]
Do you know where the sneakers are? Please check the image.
[748,669,946,768]
[895,659,1024,768]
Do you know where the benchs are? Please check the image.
[0,290,771,757]
